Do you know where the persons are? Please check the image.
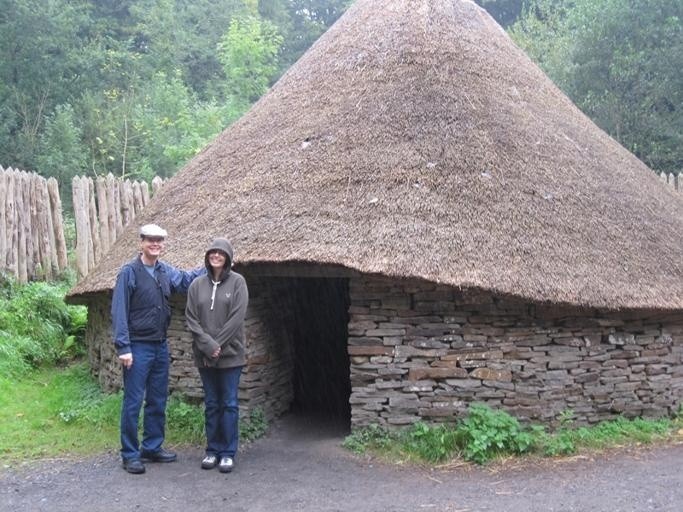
[111,224,234,473]
[184,238,249,473]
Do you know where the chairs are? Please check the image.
[202,454,234,472]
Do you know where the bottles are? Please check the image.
[140,224,167,236]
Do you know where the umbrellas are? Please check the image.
[122,447,176,473]
